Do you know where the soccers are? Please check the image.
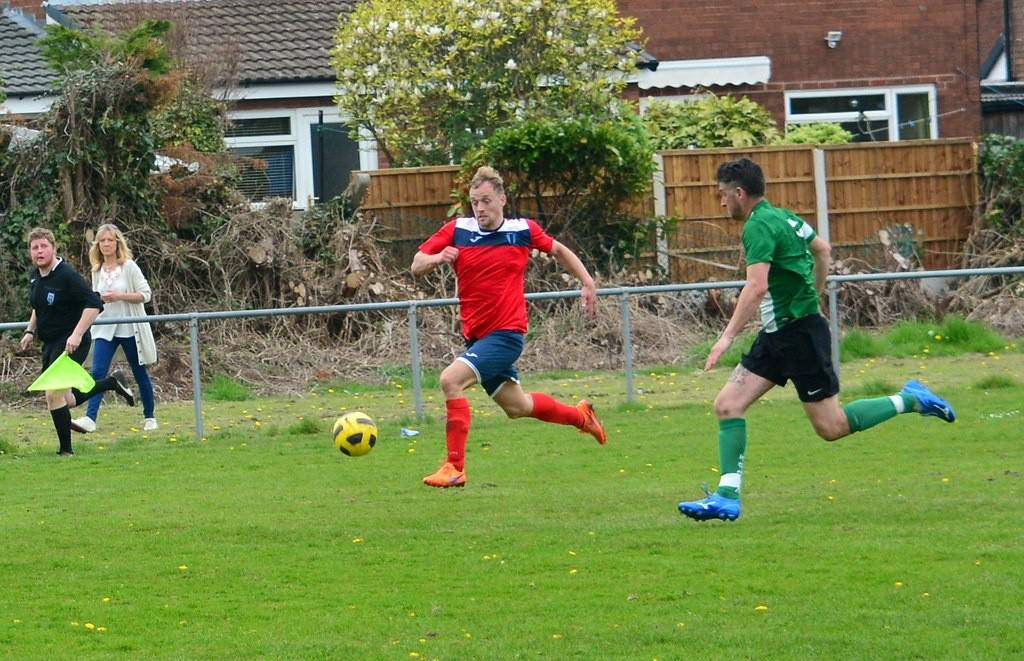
[333,411,378,456]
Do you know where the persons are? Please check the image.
[676,159,958,521]
[412,168,606,487]
[19,228,138,456]
[67,225,162,436]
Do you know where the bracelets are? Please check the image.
[23,330,35,335]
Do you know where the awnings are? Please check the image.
[642,53,773,92]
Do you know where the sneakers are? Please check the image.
[110,369,135,407]
[678,486,741,522]
[423,462,467,488]
[56,452,75,457]
[903,380,955,423]
[143,418,158,431]
[577,399,606,445]
[70,416,96,434]
